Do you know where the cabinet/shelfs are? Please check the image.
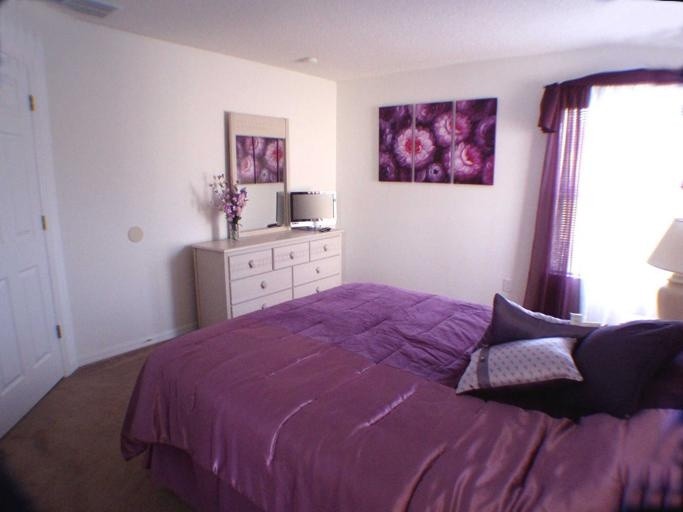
[190,227,341,330]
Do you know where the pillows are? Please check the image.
[456,291,683,409]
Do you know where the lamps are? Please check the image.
[645,218,681,319]
[292,195,333,230]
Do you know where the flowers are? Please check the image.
[210,174,247,239]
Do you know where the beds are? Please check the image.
[118,279,681,511]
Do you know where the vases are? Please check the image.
[228,221,238,243]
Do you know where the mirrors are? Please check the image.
[235,134,286,233]
